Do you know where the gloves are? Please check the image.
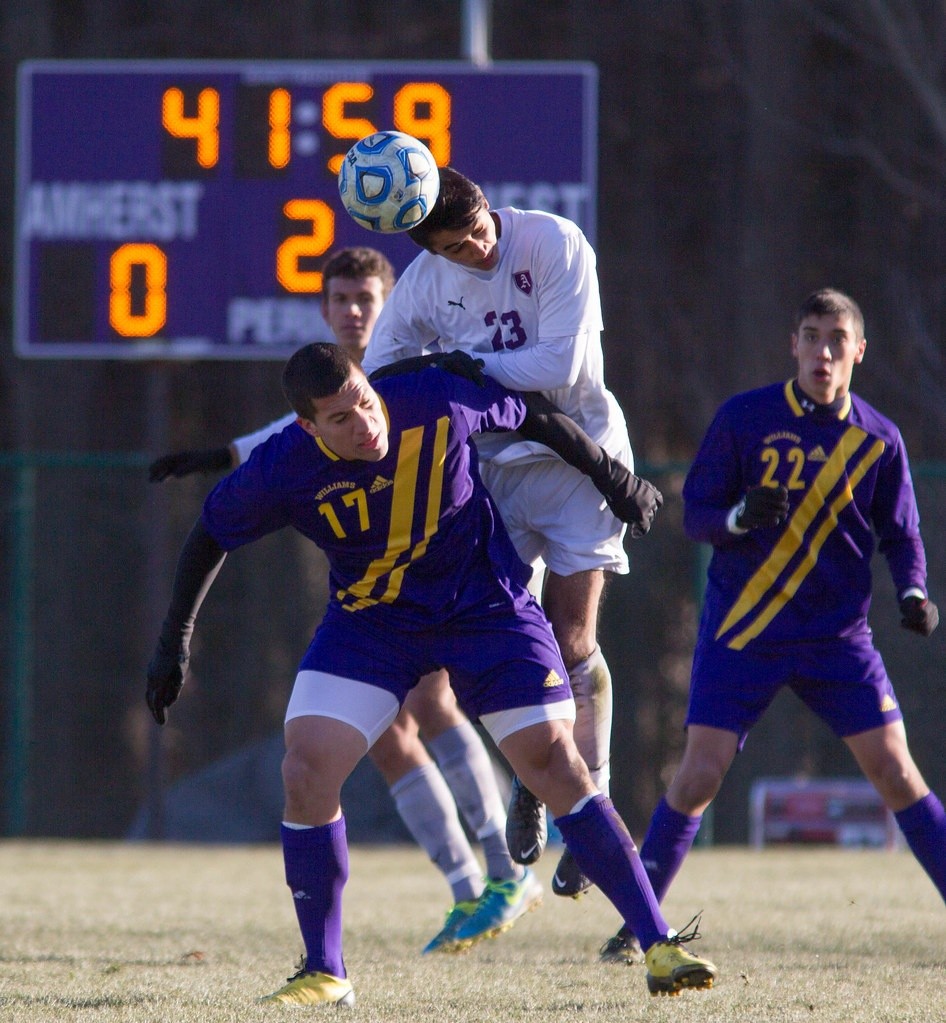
[143,635,191,724]
[147,447,234,483]
[368,349,447,381]
[439,349,489,384]
[600,456,663,535]
[735,487,791,528]
[898,594,941,637]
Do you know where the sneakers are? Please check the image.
[458,868,543,946]
[507,773,550,865]
[596,932,646,967]
[649,939,717,996]
[423,897,512,957]
[261,967,355,1009]
[553,844,597,897]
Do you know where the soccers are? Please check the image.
[338,129,438,231]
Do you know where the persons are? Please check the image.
[596,287,946,964]
[361,162,640,897]
[144,341,719,1013]
[146,245,541,952]
